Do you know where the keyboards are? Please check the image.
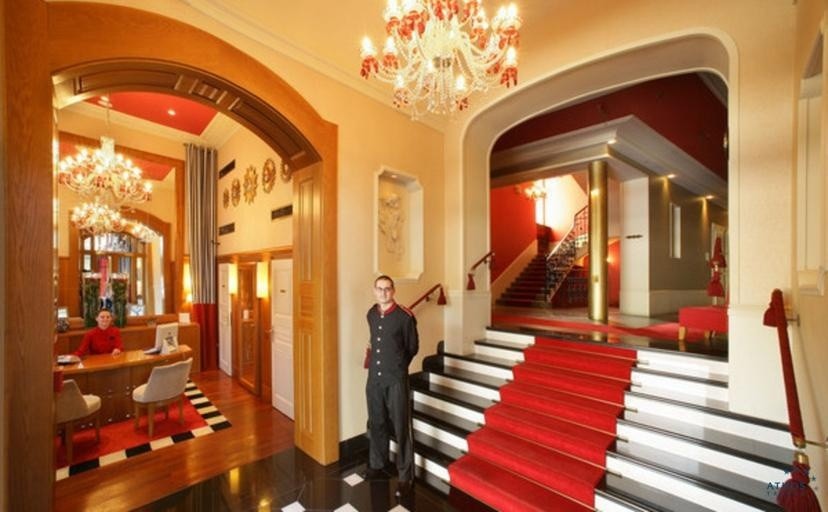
[144,349,159,355]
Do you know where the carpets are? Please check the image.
[54,377,230,483]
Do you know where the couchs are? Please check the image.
[678,305,728,348]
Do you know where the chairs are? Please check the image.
[55,380,103,466]
[131,356,194,438]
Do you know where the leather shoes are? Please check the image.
[362,467,382,479]
[395,480,413,498]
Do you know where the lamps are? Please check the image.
[56,89,154,210]
[359,0,524,123]
[68,192,157,253]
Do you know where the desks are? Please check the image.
[55,341,194,433]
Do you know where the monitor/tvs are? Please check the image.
[155,322,179,355]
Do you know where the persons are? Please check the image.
[357,274,419,498]
[67,307,122,357]
